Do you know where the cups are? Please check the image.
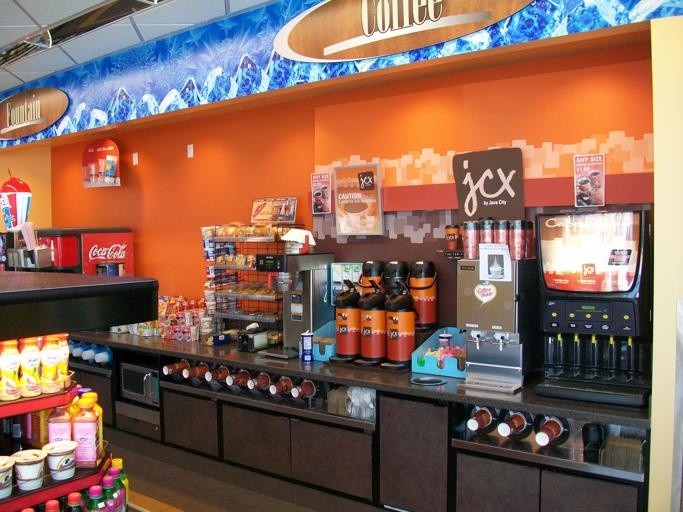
[269,376,293,395]
[0,455,16,499]
[313,185,328,205]
[343,203,368,232]
[182,362,210,379]
[42,440,79,481]
[83,154,117,185]
[577,171,602,197]
[290,380,317,399]
[247,373,271,391]
[204,365,230,382]
[497,412,528,438]
[467,407,493,431]
[534,418,564,447]
[444,225,460,252]
[0,192,32,232]
[162,359,190,376]
[9,449,48,491]
[225,369,251,387]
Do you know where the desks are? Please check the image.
[1,271,159,341]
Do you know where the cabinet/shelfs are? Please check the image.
[0,333,130,511]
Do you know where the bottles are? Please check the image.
[300,330,314,365]
[0,333,109,470]
[66,458,130,512]
[438,329,453,349]
[462,216,534,261]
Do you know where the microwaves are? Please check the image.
[118,360,164,408]
